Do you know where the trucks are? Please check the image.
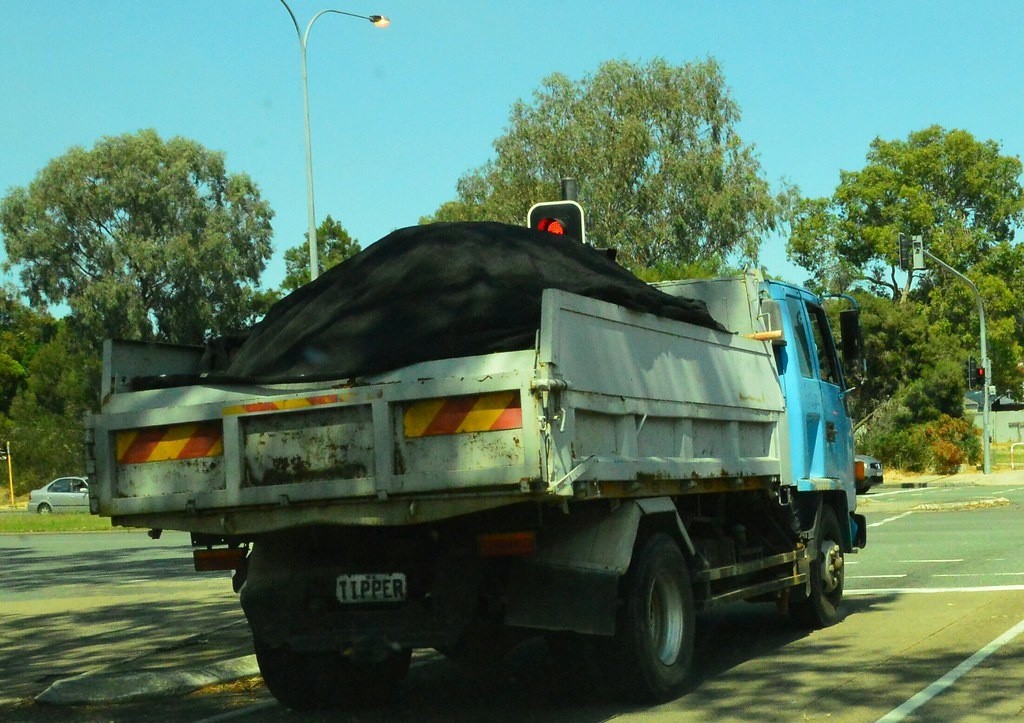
[81,275,870,723]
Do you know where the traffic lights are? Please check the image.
[526,200,587,247]
[964,355,978,392]
[977,365,987,388]
[894,233,909,270]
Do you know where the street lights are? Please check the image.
[281,0,392,281]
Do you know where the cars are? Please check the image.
[26,475,88,518]
[854,453,885,494]
